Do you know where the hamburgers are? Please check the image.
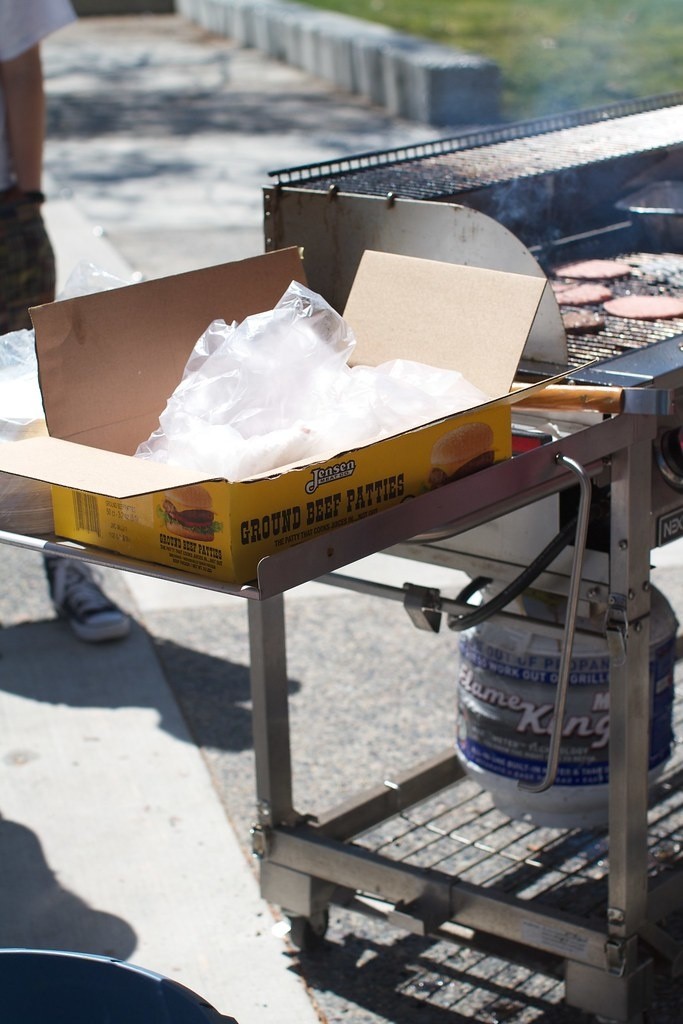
[430,422,500,490]
[156,481,223,541]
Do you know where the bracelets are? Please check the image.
[12,190,44,202]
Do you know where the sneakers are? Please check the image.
[44,556,131,642]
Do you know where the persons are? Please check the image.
[0,0,131,642]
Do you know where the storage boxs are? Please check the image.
[0,247,601,583]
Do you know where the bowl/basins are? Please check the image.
[614,181,683,254]
